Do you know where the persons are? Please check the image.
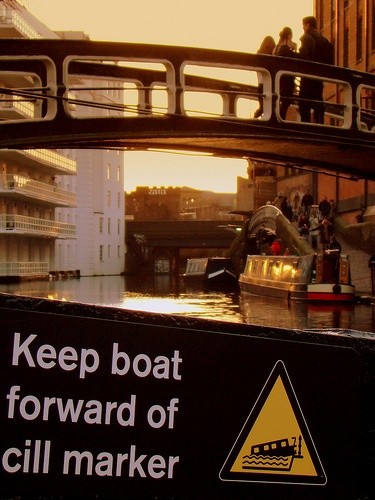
[368,254,375,297]
[265,188,342,256]
[252,35,277,120]
[271,27,297,121]
[298,16,335,123]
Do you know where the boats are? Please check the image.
[238,253,357,303]
[178,256,238,292]
